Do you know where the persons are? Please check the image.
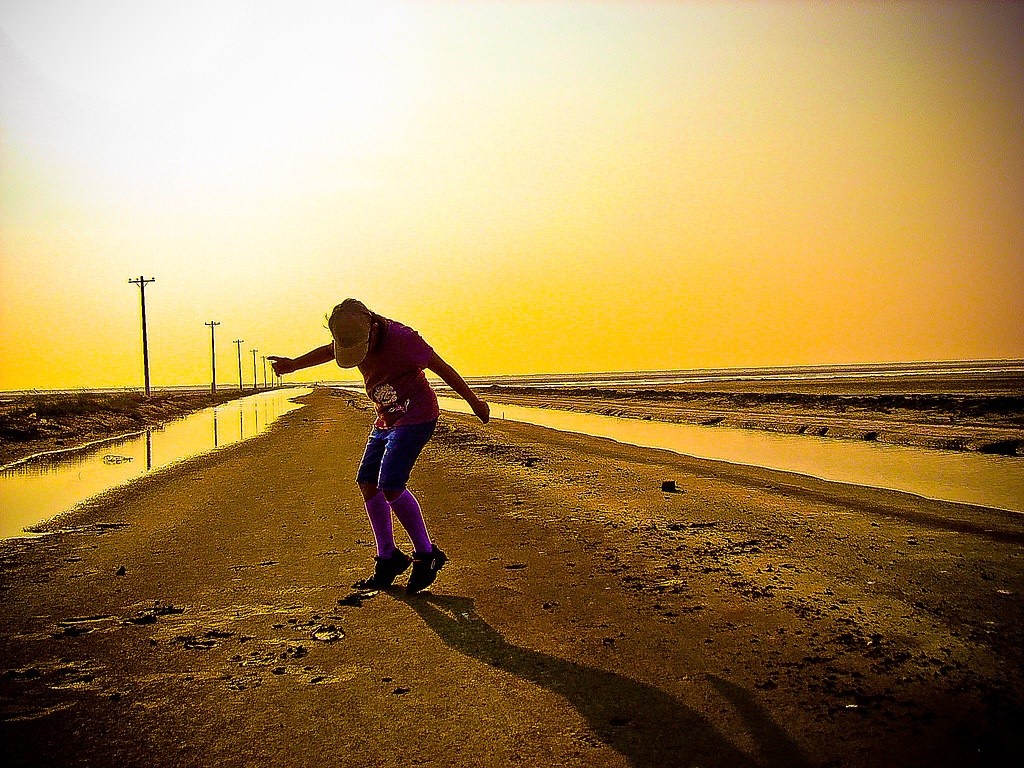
[265,298,492,593]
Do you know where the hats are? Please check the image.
[328,297,371,369]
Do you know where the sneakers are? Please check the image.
[365,548,412,589]
[407,544,447,591]
